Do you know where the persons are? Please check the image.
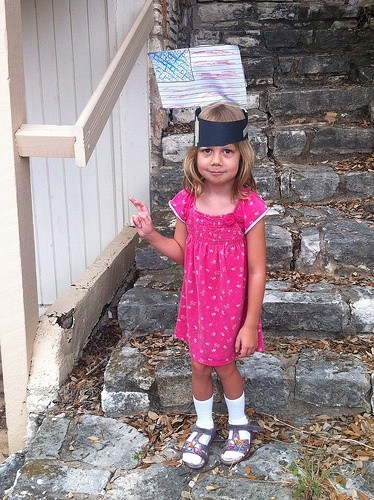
[130,103,274,469]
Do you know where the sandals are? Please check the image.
[220,414,253,465]
[183,421,219,469]
